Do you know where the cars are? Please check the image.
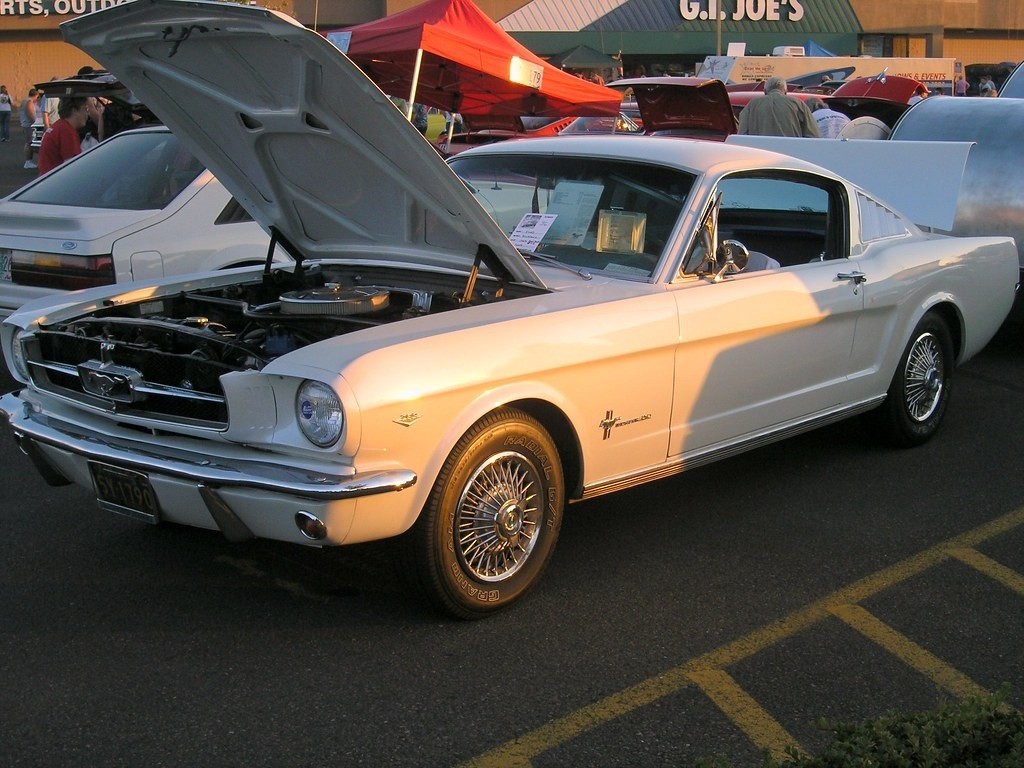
[27,68,166,165]
[0,1,1020,617]
[442,74,933,190]
[0,117,555,309]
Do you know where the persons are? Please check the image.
[739,75,820,138]
[19,88,39,169]
[956,75,970,96]
[405,101,428,136]
[803,97,851,138]
[39,97,109,178]
[41,76,62,133]
[906,87,945,106]
[439,109,463,135]
[0,85,14,143]
[978,75,998,97]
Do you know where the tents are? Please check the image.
[544,44,623,85]
[315,0,624,155]
